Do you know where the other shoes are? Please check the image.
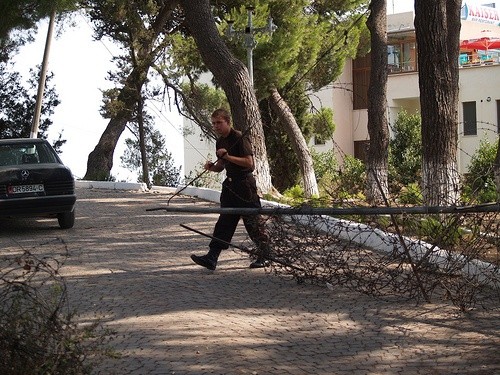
[249,258,270,268]
[190,249,221,271]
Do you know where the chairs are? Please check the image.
[22,154,38,164]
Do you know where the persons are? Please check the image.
[190,109,274,271]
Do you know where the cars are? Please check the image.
[0,137,77,228]
